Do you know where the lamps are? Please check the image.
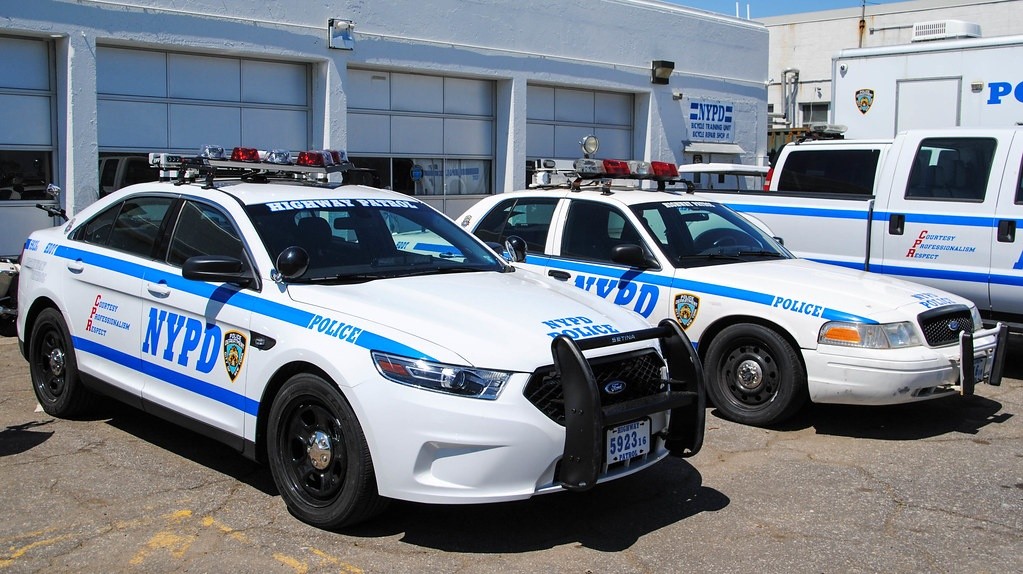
[652,61,675,85]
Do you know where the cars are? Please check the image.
[519,135,1008,428]
[15,146,708,530]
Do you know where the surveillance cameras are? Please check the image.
[840,64,848,72]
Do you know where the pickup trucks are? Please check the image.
[664,126,1023,335]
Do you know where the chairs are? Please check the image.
[619,216,648,243]
[296,217,338,266]
[936,160,976,196]
[593,216,608,242]
[832,163,869,193]
